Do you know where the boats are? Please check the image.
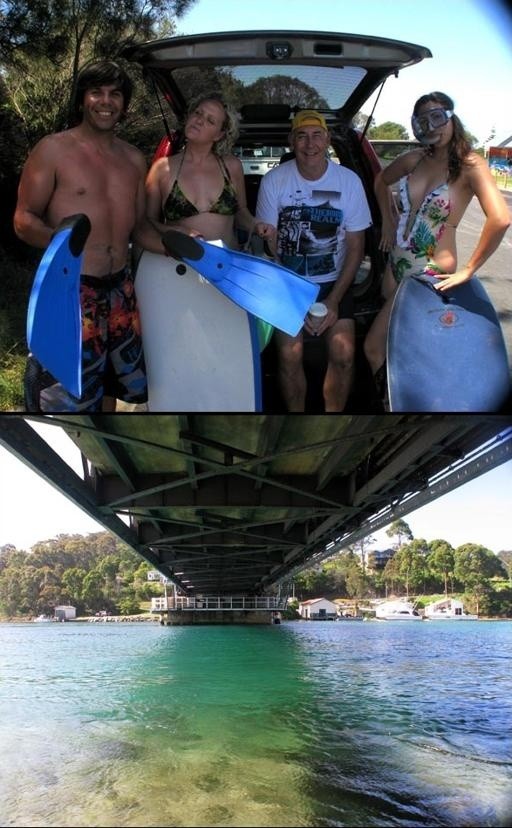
[381,591,428,620]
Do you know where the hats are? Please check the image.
[291,109,328,132]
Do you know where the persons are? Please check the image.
[13,60,512,413]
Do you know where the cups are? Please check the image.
[310,303,327,330]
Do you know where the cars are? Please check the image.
[286,597,298,603]
[94,610,113,617]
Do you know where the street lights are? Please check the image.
[292,575,296,599]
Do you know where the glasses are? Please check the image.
[412,108,454,137]
